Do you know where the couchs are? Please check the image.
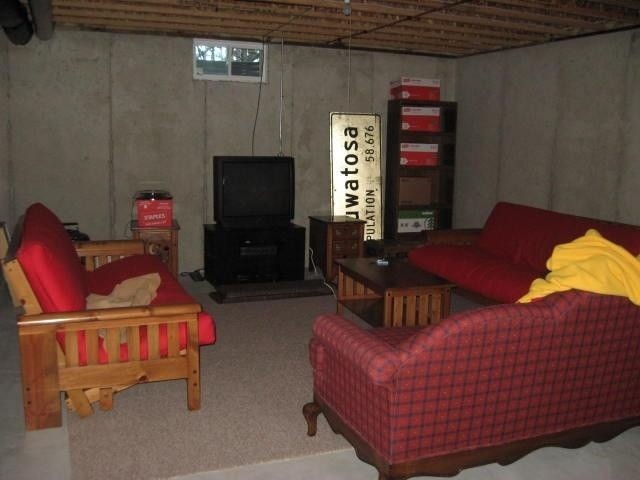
[1,202,217,432]
[302,287,640,479]
[408,200,640,306]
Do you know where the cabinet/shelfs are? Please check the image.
[202,224,306,289]
[384,98,458,240]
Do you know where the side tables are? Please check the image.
[130,220,180,281]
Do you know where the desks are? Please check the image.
[307,213,368,280]
[331,257,458,331]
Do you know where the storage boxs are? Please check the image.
[397,204,437,234]
[390,76,443,168]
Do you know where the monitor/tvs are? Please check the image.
[213,155,295,230]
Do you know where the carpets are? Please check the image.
[65,269,488,480]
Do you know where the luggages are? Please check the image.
[62,223,89,241]
